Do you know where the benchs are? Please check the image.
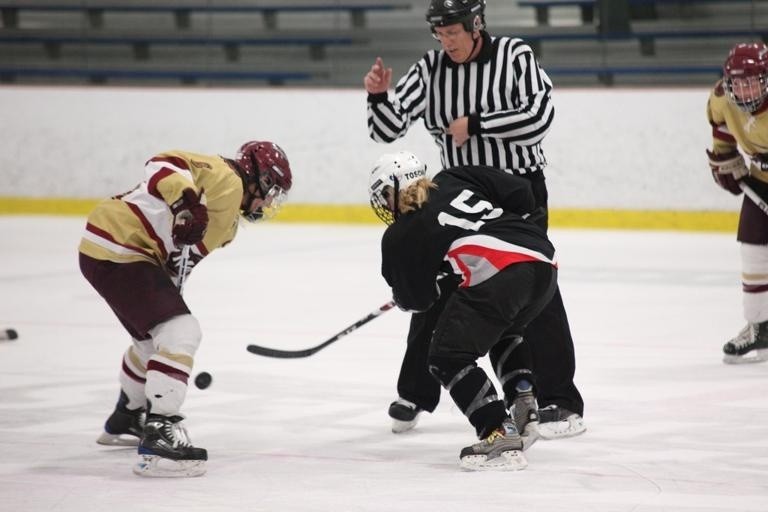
[0,0,735,91]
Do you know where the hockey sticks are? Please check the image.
[247,300,397,358]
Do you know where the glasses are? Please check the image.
[431,31,465,40]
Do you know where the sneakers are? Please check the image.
[724,322,768,354]
[389,396,423,421]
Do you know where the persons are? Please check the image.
[364,1,583,425]
[707,41,767,356]
[79,139,292,460]
[371,153,558,458]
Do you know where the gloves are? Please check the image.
[706,148,749,194]
[169,185,208,251]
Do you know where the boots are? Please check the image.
[138,398,207,460]
[460,378,580,460]
[105,389,147,438]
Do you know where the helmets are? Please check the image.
[425,0,486,41]
[369,151,427,225]
[722,41,768,115]
[221,141,292,223]
